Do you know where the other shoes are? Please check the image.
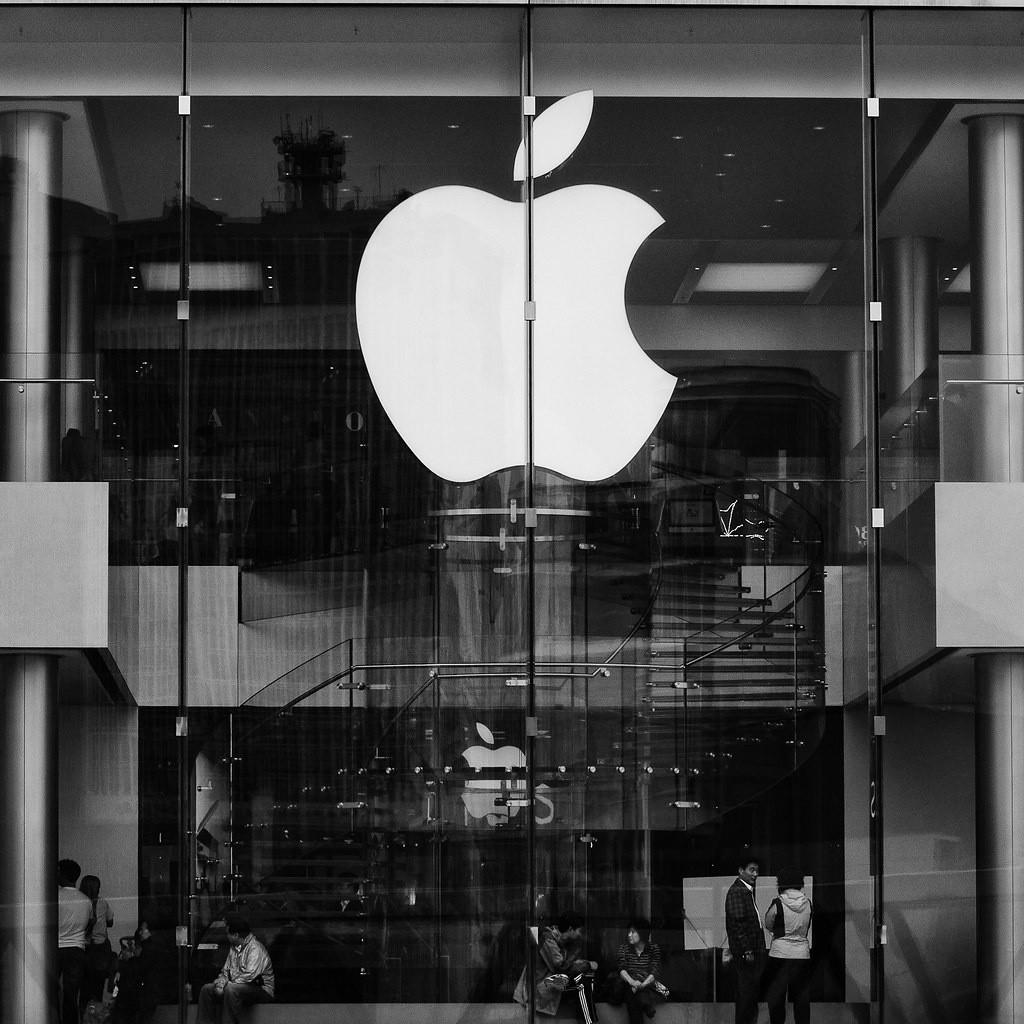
[544,976,569,992]
[645,1008,656,1019]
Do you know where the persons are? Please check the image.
[615,917,660,1024]
[61,426,335,569]
[764,866,814,1024]
[195,916,276,1024]
[513,911,599,1024]
[56,858,115,1024]
[725,856,766,1024]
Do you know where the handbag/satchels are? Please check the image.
[82,999,114,1024]
